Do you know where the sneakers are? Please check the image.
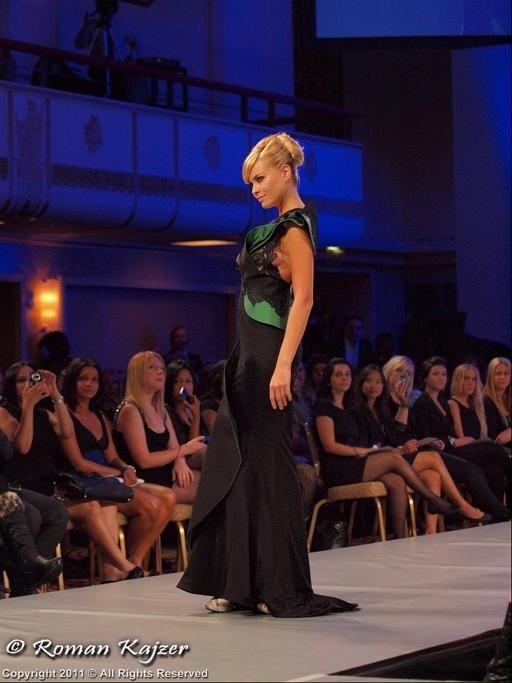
[205,597,270,614]
[426,498,512,523]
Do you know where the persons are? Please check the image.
[163,327,188,363]
[1,349,511,602]
[175,129,361,619]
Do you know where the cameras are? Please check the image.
[29,371,43,388]
[399,371,409,384]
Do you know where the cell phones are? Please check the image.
[179,386,191,403]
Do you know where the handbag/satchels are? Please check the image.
[39,467,134,504]
[312,520,347,550]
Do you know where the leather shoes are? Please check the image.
[101,566,159,583]
[28,555,64,588]
